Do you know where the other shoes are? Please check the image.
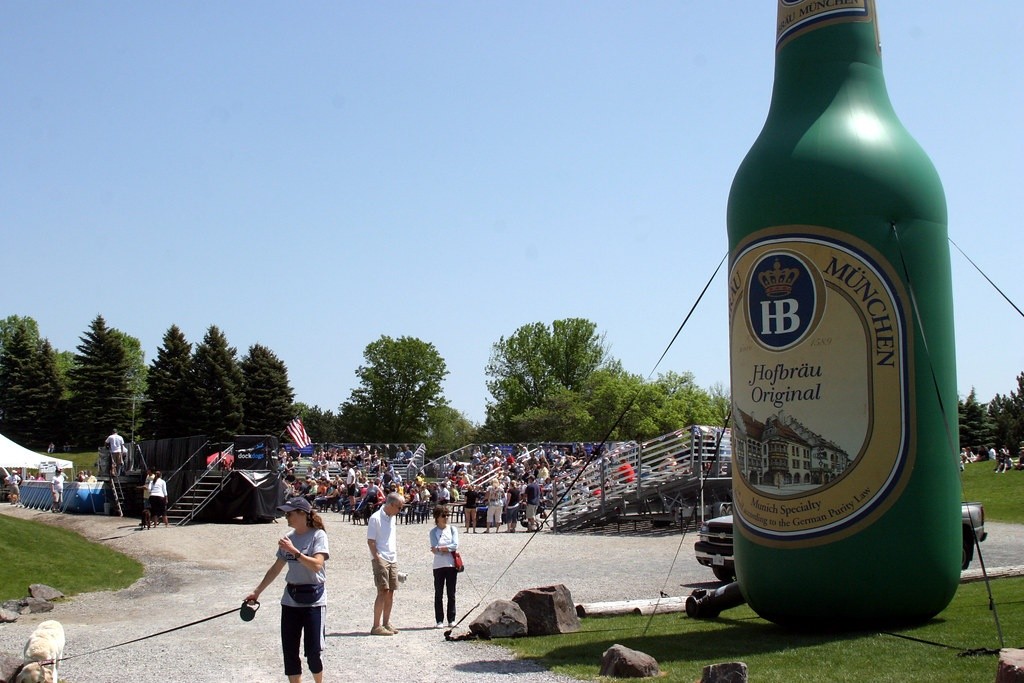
[464,526,538,533]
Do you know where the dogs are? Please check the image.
[139,509,151,530]
[16,620,65,683]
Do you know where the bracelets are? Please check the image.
[437,547,439,552]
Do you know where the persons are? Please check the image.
[404,472,459,511]
[47,442,55,453]
[430,505,458,629]
[104,428,125,476]
[63,442,71,452]
[443,445,610,533]
[0,469,97,513]
[139,468,171,527]
[221,457,234,470]
[608,434,716,487]
[367,492,405,636]
[243,498,331,683]
[278,446,413,512]
[959,441,1024,474]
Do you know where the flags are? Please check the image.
[286,415,312,448]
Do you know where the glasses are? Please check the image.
[394,505,402,511]
[284,510,301,516]
[437,513,451,518]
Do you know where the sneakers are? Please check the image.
[447,622,460,628]
[436,622,444,629]
[385,624,398,633]
[370,626,393,636]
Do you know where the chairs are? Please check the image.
[314,496,466,526]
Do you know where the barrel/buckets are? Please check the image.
[725,0,964,638]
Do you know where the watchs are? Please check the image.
[295,553,300,558]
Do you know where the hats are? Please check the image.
[13,470,18,474]
[276,498,312,513]
[526,475,536,480]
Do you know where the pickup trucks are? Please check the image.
[693,498,989,584]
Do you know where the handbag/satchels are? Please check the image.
[286,583,325,605]
[452,552,464,573]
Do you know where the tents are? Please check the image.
[207,452,234,464]
[0,434,73,482]
